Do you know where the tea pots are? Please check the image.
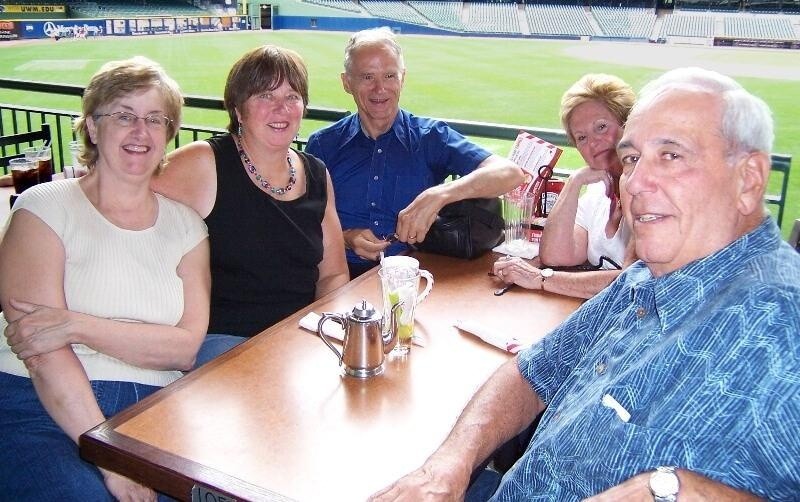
[318,300,405,382]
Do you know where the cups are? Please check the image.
[24,146,52,185]
[9,158,39,194]
[503,191,535,252]
[68,140,89,177]
[377,254,435,355]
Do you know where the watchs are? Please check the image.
[647,465,680,502]
[540,268,552,294]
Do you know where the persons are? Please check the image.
[148,46,351,370]
[365,69,800,502]
[46,25,98,42]
[0,56,211,502]
[490,74,639,303]
[306,27,526,278]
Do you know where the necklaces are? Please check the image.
[611,192,622,210]
[236,139,297,195]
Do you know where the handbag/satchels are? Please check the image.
[408,195,506,260]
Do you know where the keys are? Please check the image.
[381,232,400,245]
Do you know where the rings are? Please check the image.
[500,268,505,280]
[408,236,417,240]
[505,255,510,260]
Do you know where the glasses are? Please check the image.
[92,111,175,131]
